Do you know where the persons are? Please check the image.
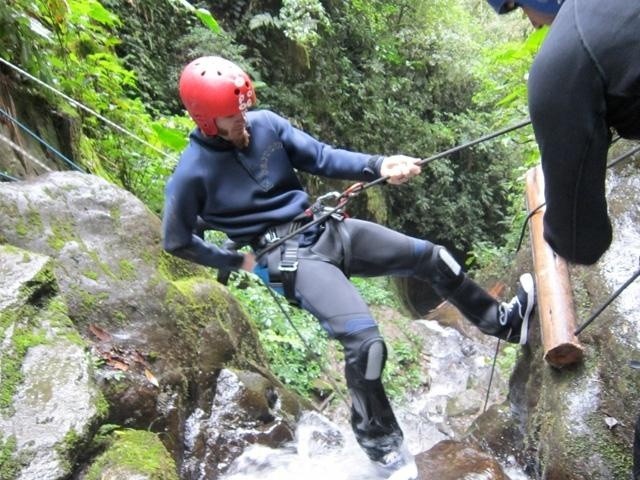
[163,56,535,479]
[486,0,640,264]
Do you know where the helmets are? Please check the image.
[178,56,257,136]
[485,0,565,16]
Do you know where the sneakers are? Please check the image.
[369,444,419,480]
[502,272,535,345]
